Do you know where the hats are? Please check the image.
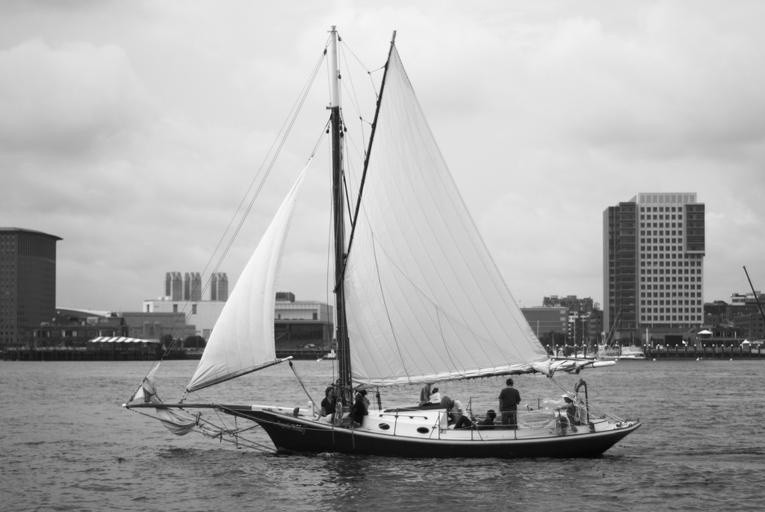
[561,391,575,402]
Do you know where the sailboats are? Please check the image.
[595,309,647,359]
[121,25,643,458]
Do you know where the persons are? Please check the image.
[499,378,521,425]
[441,396,496,429]
[553,393,587,429]
[420,384,441,405]
[312,386,368,428]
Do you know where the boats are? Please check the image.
[322,348,338,361]
